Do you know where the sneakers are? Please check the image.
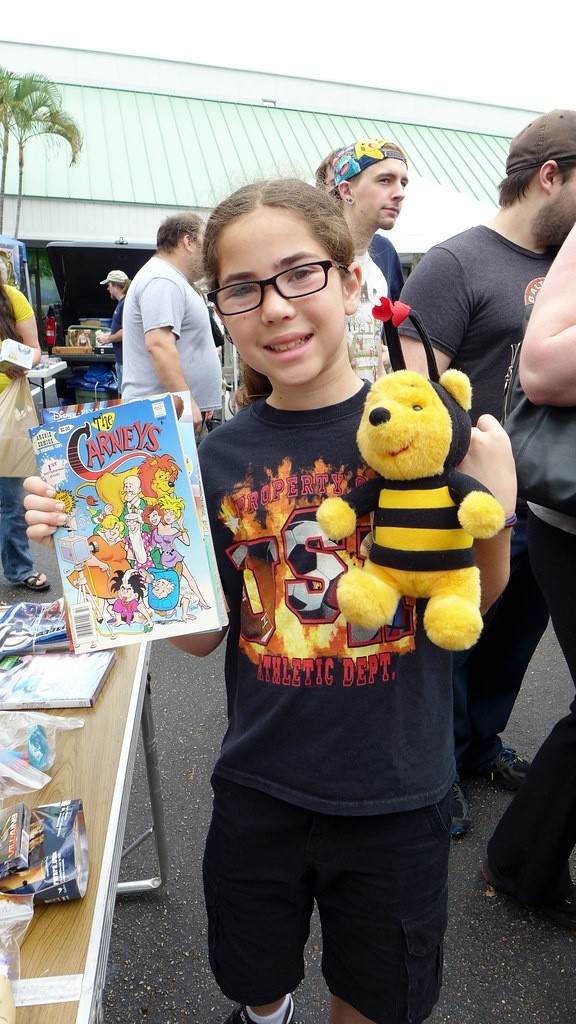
[221,992,294,1024]
[462,743,530,790]
[449,784,472,839]
[480,854,576,924]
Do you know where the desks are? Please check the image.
[27,360,68,409]
[0,605,170,1024]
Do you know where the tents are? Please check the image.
[0,234,31,302]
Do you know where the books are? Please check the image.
[0,597,118,709]
[28,390,230,655]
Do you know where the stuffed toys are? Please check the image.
[316,297,506,651]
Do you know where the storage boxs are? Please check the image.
[52,318,113,353]
[0,338,34,373]
[74,388,114,403]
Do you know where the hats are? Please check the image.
[325,139,408,194]
[100,270,128,285]
[506,110,576,176]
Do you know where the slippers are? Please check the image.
[22,572,50,589]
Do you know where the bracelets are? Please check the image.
[505,514,517,528]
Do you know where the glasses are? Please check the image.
[207,260,351,316]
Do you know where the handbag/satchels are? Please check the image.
[501,342,576,516]
[0,376,42,478]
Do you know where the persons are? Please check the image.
[315,147,341,200]
[0,266,51,590]
[327,138,409,382]
[21,177,518,1024]
[97,270,132,399]
[120,212,223,435]
[481,224,576,927]
[397,107,576,835]
